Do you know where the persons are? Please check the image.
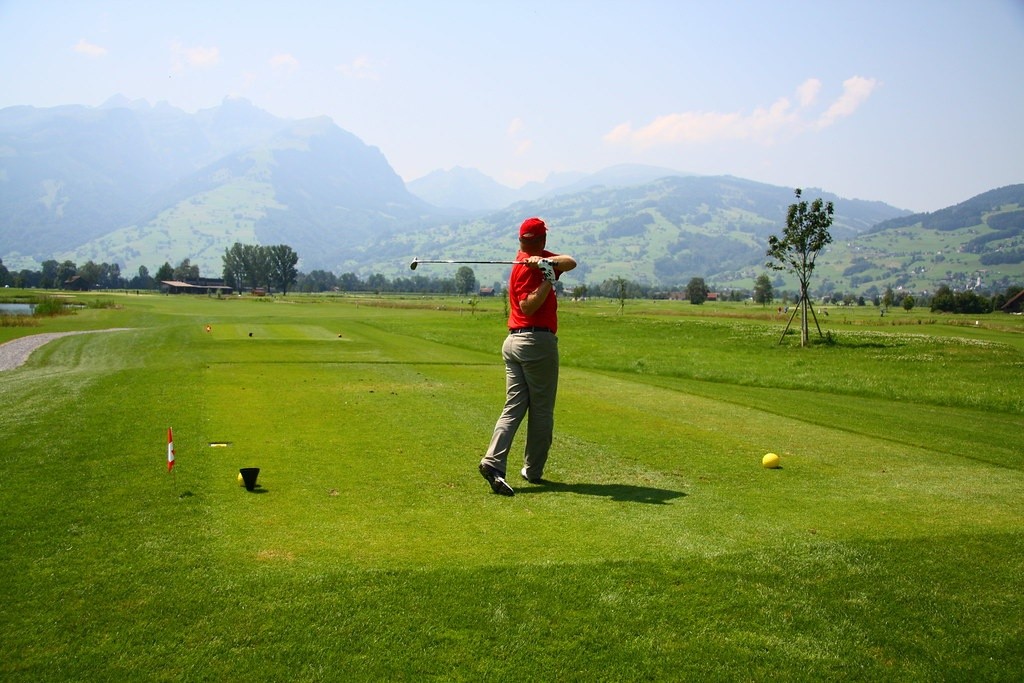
[478,218,579,499]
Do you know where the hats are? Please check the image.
[520,218,548,240]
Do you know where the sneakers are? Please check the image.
[479,463,514,496]
[521,468,541,484]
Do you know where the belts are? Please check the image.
[511,326,553,333]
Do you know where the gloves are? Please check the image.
[538,258,557,295]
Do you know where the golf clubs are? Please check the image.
[409,256,559,271]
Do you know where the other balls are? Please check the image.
[762,452,780,469]
[237,472,258,486]
[338,333,342,337]
